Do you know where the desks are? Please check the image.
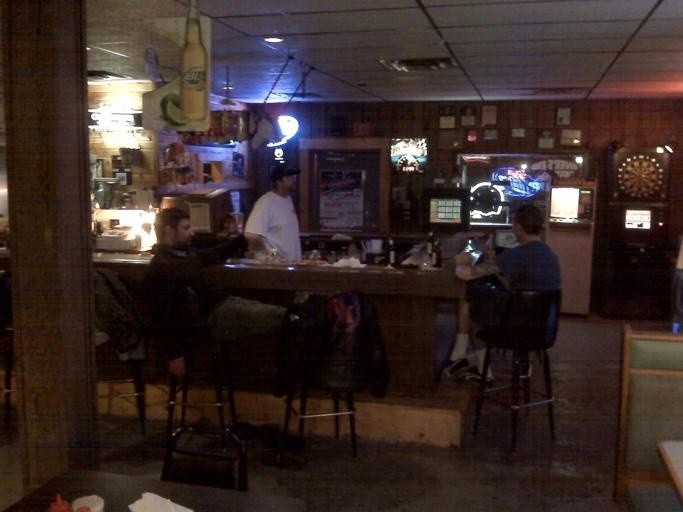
[2,469,307,511]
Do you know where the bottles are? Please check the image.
[386,237,397,267]
[309,249,320,268]
[181,2,208,121]
[427,230,442,268]
[217,211,249,264]
[94,181,120,208]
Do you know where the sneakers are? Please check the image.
[438,358,474,379]
[456,367,494,386]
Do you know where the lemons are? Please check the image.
[166,101,186,125]
[160,92,180,114]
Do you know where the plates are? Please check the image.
[102,229,126,238]
[112,225,133,231]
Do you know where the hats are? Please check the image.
[270,165,300,179]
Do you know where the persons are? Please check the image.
[439,202,561,395]
[141,207,355,349]
[242,164,303,260]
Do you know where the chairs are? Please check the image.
[92,268,563,492]
[613,323,682,511]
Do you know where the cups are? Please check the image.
[93,221,103,238]
[109,218,120,229]
[672,323,680,334]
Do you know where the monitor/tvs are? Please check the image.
[622,207,654,231]
[427,193,466,226]
[549,186,580,219]
